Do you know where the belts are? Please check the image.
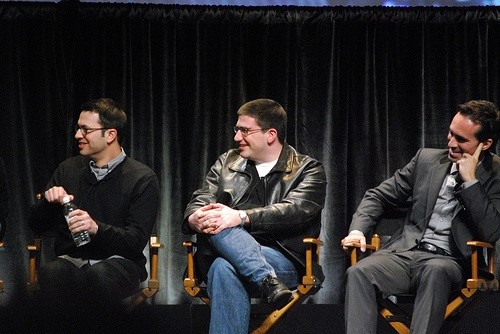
[414,242,451,257]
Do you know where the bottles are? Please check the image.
[62,197,89,247]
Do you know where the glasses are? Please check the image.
[233,125,264,137]
[73,123,108,136]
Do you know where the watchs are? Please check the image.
[239,210,247,227]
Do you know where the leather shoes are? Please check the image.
[262,274,294,311]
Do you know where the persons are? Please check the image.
[181,98,328,334]
[342,100,500,334]
[27,98,161,308]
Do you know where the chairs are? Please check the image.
[345,234,499,334]
[183,236,324,334]
[26,194,164,316]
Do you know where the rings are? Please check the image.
[82,220,84,225]
